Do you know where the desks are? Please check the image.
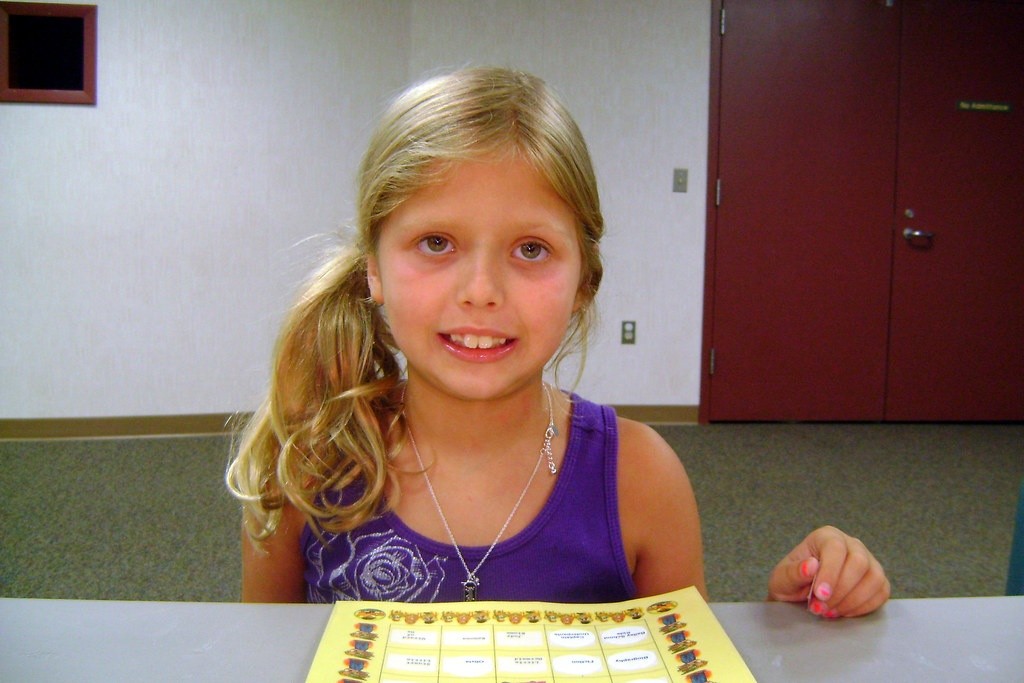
[0,595,1024,683]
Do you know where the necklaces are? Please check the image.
[398,376,557,601]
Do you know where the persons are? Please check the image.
[216,57,893,618]
